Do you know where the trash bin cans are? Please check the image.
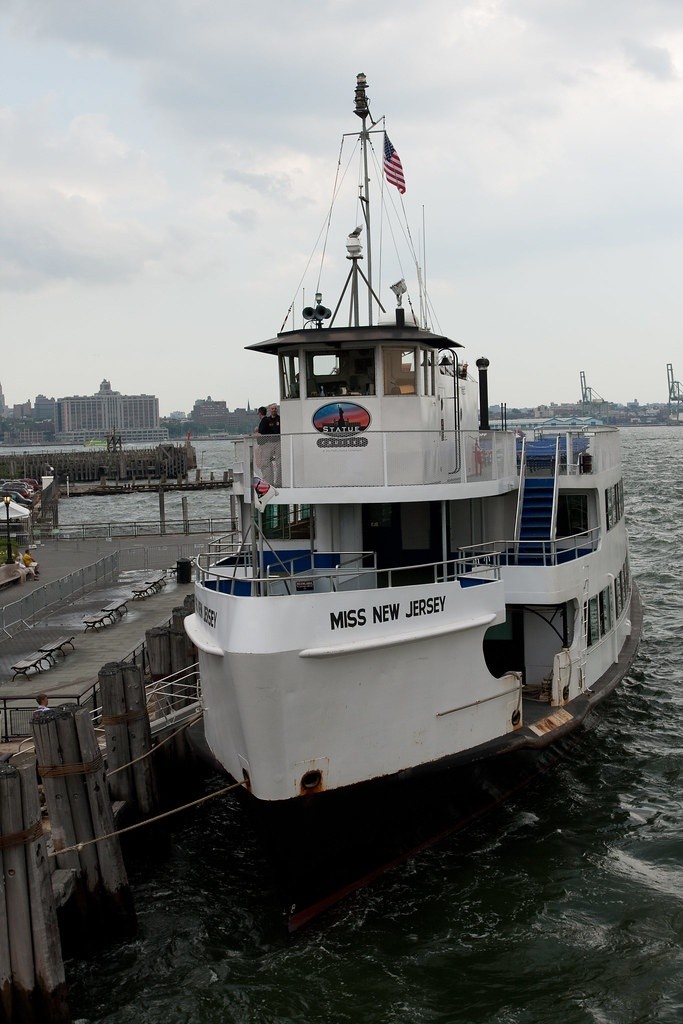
[177,558,191,583]
[582,454,592,473]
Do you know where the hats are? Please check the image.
[259,407,267,412]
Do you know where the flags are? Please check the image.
[383,133,406,194]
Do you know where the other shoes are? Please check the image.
[34,578,39,580]
[34,575,38,577]
[35,571,39,574]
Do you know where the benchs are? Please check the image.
[169,557,196,577]
[83,599,128,633]
[132,575,167,601]
[0,563,29,590]
[11,635,75,682]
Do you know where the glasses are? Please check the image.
[272,408,277,410]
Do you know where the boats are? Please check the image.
[182,73,643,941]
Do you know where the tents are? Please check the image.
[0,501,32,549]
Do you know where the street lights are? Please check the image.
[201,450,206,468]
[2,492,16,564]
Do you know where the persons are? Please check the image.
[34,693,51,714]
[255,403,283,488]
[14,549,40,584]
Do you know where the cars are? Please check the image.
[0,479,39,508]
